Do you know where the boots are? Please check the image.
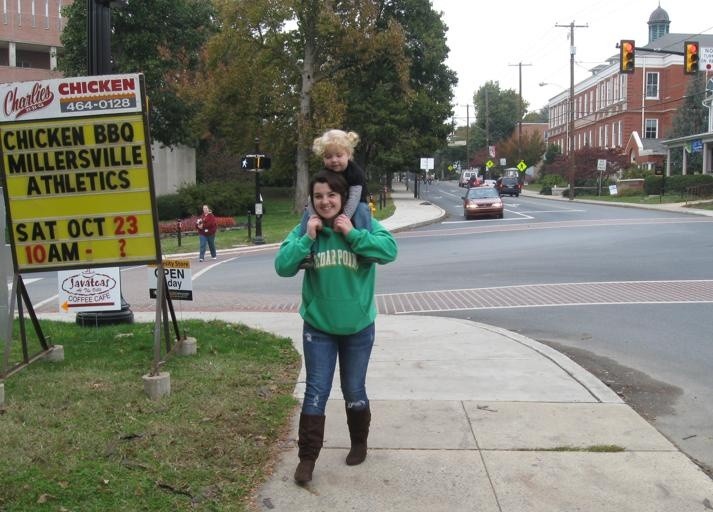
[294,412,327,484]
[345,401,374,466]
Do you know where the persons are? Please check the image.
[273,169,399,481]
[195,204,217,262]
[298,130,378,269]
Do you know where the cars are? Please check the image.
[461,186,504,220]
[480,179,496,188]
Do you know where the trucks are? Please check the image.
[458,171,477,187]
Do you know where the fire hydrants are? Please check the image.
[367,201,375,217]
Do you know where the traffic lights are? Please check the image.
[259,158,271,169]
[239,157,255,170]
[685,42,699,75]
[655,167,663,175]
[619,40,635,74]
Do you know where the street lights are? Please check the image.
[536,82,574,200]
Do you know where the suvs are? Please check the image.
[493,177,519,198]
[467,177,482,188]
